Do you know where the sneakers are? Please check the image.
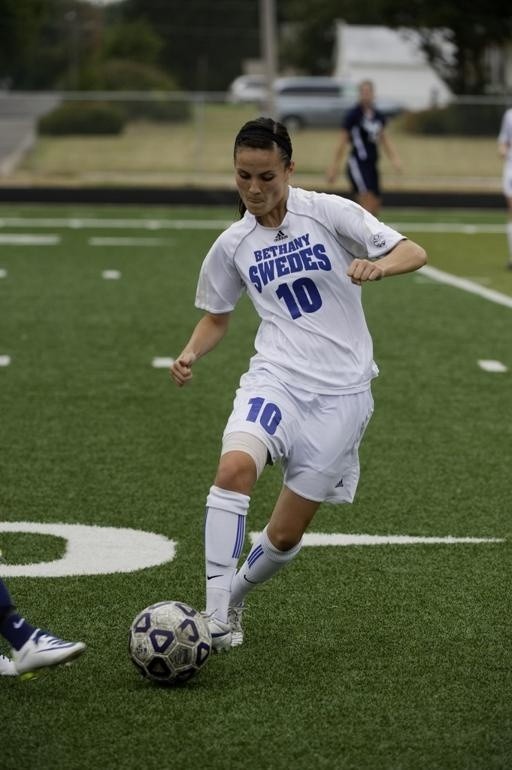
[0,654,21,677]
[199,610,233,653]
[227,601,247,648]
[8,627,88,675]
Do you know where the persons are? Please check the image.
[0,584,85,677]
[330,79,402,218]
[170,118,426,650]
[498,110,511,272]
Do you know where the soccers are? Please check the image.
[128,600,211,685]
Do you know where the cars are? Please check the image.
[231,73,269,107]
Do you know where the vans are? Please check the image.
[274,77,410,133]
